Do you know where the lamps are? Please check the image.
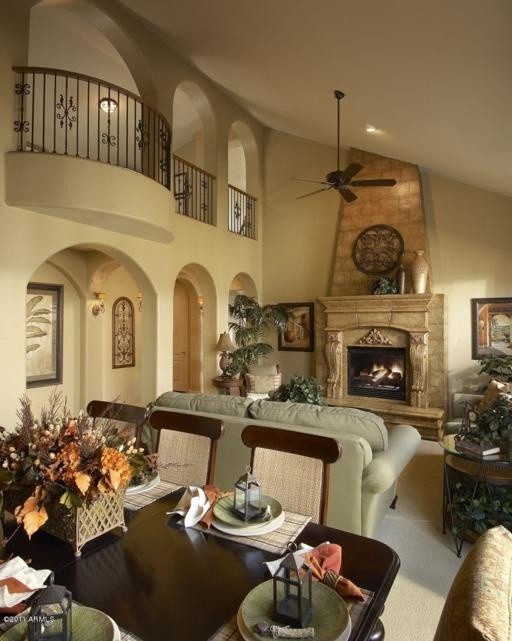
[198,295,204,314]
[136,290,143,311]
[99,97,117,113]
[93,289,106,316]
[215,330,235,377]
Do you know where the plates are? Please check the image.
[209,508,286,538]
[212,492,282,528]
[125,470,162,496]
[241,577,349,641]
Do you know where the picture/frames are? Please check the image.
[25,280,64,388]
[470,296,512,360]
[111,295,135,369]
[175,173,188,198]
[277,301,317,353]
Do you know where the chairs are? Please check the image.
[244,362,281,401]
[240,424,343,527]
[445,392,486,434]
[86,399,148,449]
[433,525,512,641]
[150,410,226,489]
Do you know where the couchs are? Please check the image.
[150,391,422,538]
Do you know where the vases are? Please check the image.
[397,264,406,294]
[409,249,429,293]
[40,490,129,557]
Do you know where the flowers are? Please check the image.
[0,386,131,541]
[369,274,398,295]
[447,481,511,534]
[283,375,326,406]
[478,356,512,381]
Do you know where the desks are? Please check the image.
[439,432,512,559]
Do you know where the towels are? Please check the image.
[165,485,238,530]
[0,555,51,613]
[263,542,364,602]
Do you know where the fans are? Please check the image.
[289,160,396,203]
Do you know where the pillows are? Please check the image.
[245,373,281,394]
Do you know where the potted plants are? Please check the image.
[228,294,288,388]
